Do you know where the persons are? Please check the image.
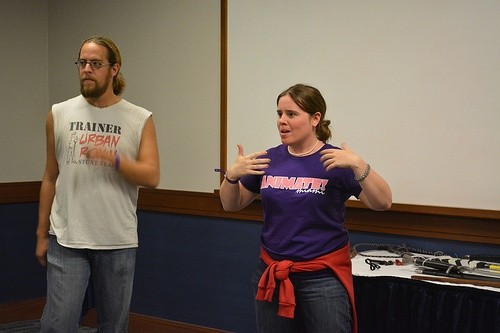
[219,83,393,333]
[36,36,161,333]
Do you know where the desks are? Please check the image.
[351,249,500,333]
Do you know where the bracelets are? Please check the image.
[224,174,240,184]
[355,164,370,182]
[115,153,120,170]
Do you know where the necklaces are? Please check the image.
[288,140,320,155]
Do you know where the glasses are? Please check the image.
[74,60,114,69]
